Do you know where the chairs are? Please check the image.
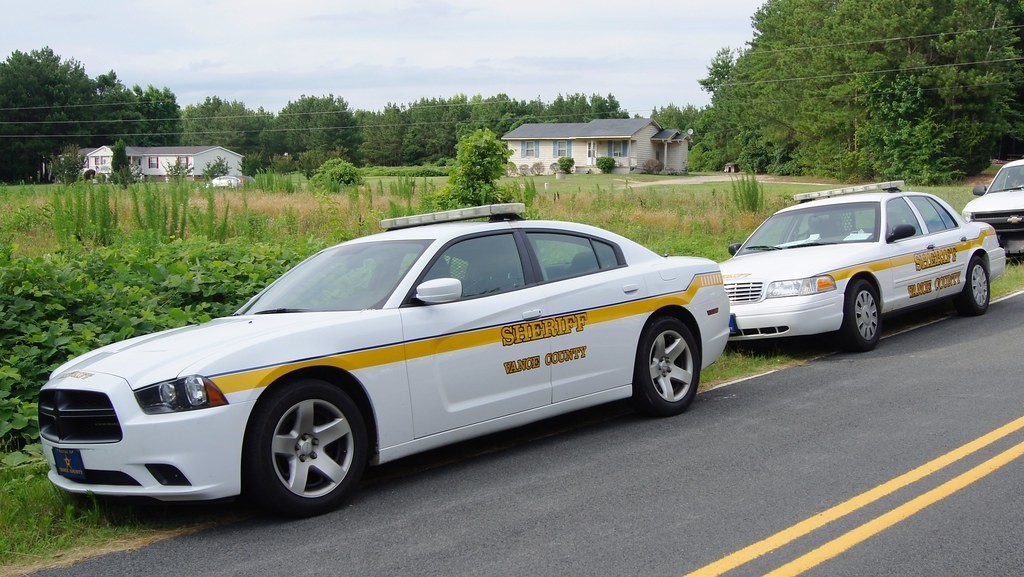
[419,258,451,282]
[807,219,842,238]
[569,252,603,274]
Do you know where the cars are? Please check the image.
[203,175,258,190]
[39,205,730,516]
[961,160,1024,262]
[719,178,1007,355]
